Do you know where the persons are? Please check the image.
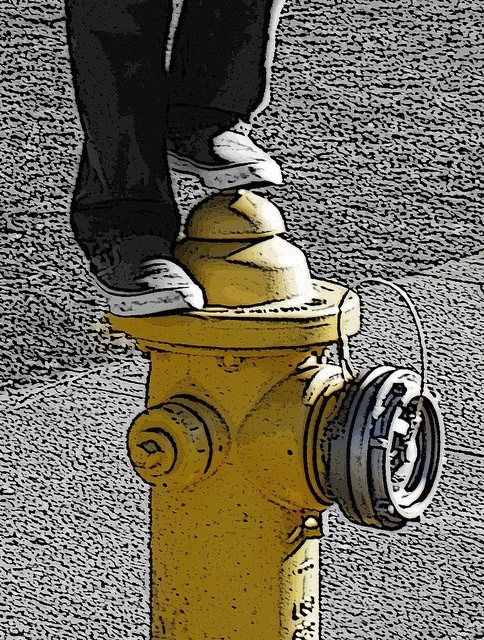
[60,1,284,316]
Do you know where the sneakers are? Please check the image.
[84,232,205,318]
[163,116,284,191]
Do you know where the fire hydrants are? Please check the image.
[107,189,444,640]
